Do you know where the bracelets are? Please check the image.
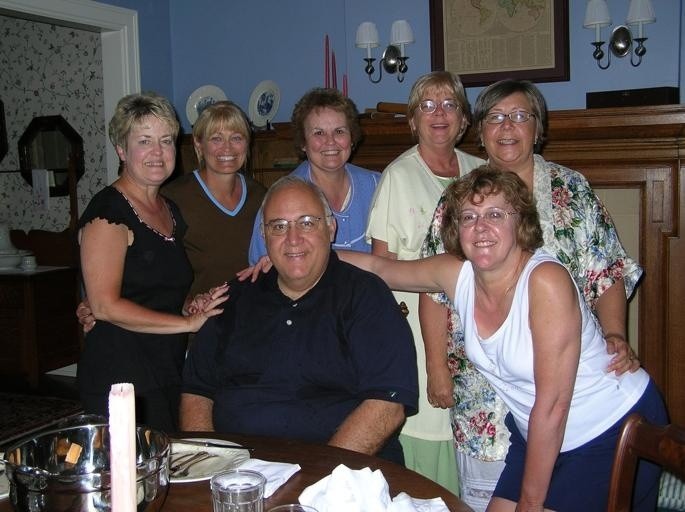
[603,332,626,341]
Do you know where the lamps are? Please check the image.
[354,20,415,84]
[583,0,657,70]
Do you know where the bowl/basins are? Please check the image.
[0,253,30,271]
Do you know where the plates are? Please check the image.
[170,438,251,484]
[185,85,228,126]
[247,79,281,128]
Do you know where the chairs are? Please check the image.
[607,412,685,512]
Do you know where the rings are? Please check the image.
[629,355,636,361]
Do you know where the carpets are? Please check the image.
[0,385,84,454]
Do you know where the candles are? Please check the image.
[108,382,137,512]
[325,34,347,96]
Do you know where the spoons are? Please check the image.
[170,453,219,480]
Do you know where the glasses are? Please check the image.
[484,110,537,124]
[458,207,518,228]
[413,99,461,114]
[264,215,333,236]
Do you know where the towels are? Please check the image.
[298,463,450,512]
[210,458,301,498]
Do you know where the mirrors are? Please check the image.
[17,115,85,197]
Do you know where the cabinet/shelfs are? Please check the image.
[0,267,80,387]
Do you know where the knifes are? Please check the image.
[169,439,257,450]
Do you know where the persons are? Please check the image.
[247,86,383,266]
[196,98,215,118]
[417,78,645,512]
[364,67,490,499]
[257,94,274,116]
[236,162,670,510]
[77,90,230,434]
[76,100,268,362]
[171,175,418,471]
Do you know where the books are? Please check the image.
[357,102,416,120]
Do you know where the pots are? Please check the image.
[0,424,170,512]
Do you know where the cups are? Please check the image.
[20,256,37,272]
[268,504,319,512]
[210,469,267,512]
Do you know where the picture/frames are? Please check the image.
[429,0,570,88]
